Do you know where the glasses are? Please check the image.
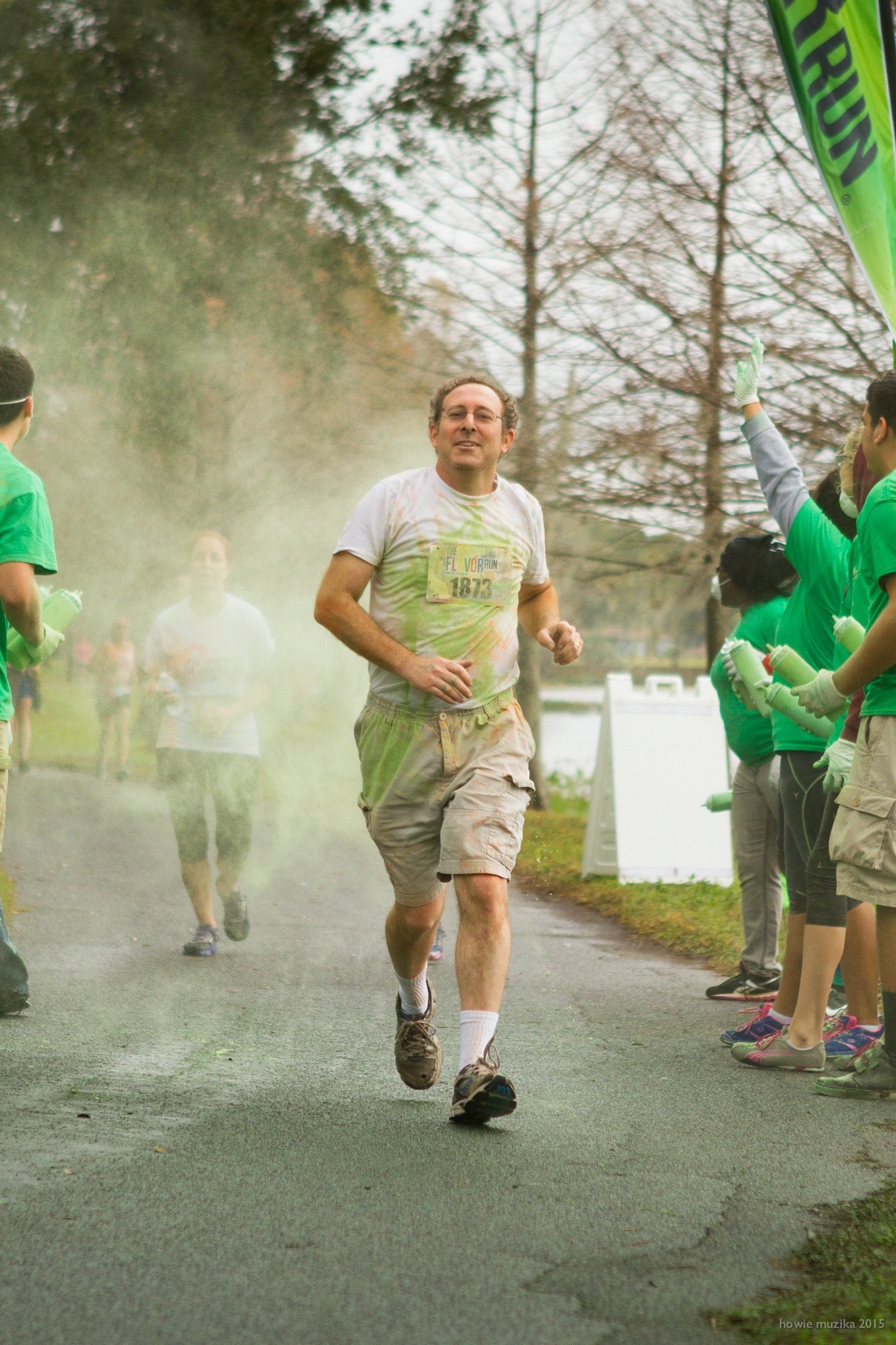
[834,453,851,470]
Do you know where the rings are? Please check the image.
[574,642,580,648]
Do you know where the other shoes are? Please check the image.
[427,920,447,960]
[823,1005,849,1027]
[183,924,220,957]
[215,875,251,941]
[0,908,31,1015]
[822,1024,885,1057]
[821,1014,857,1046]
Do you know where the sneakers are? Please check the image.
[705,959,782,1001]
[719,1003,785,1045]
[395,975,443,1090]
[831,1038,896,1071]
[812,1048,896,1101]
[448,1029,518,1125]
[730,1024,826,1072]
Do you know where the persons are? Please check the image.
[6,662,42,777]
[0,346,57,1016]
[313,378,586,1121]
[83,528,272,959]
[705,336,896,1101]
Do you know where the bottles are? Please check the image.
[831,615,866,652]
[765,644,850,722]
[760,682,836,741]
[700,790,734,811]
[720,635,773,718]
[5,583,85,673]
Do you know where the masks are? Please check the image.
[839,488,859,519]
[710,575,722,602]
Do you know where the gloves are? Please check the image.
[730,674,773,704]
[734,336,764,409]
[24,623,65,666]
[813,738,857,794]
[791,668,849,720]
[720,645,767,682]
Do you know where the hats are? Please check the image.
[719,533,797,602]
